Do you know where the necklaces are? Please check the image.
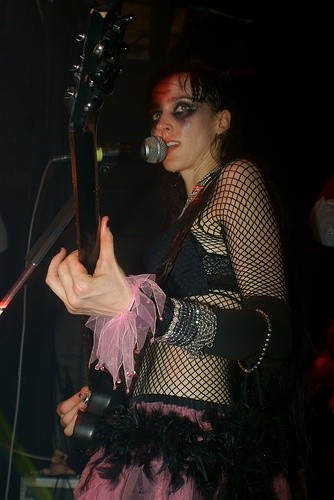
[177,163,224,221]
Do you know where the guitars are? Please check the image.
[62,1,135,482]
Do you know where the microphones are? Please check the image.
[97,135,168,164]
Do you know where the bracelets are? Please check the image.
[237,307,273,374]
[84,273,166,394]
[160,297,219,356]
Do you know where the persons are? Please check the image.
[45,54,298,500]
